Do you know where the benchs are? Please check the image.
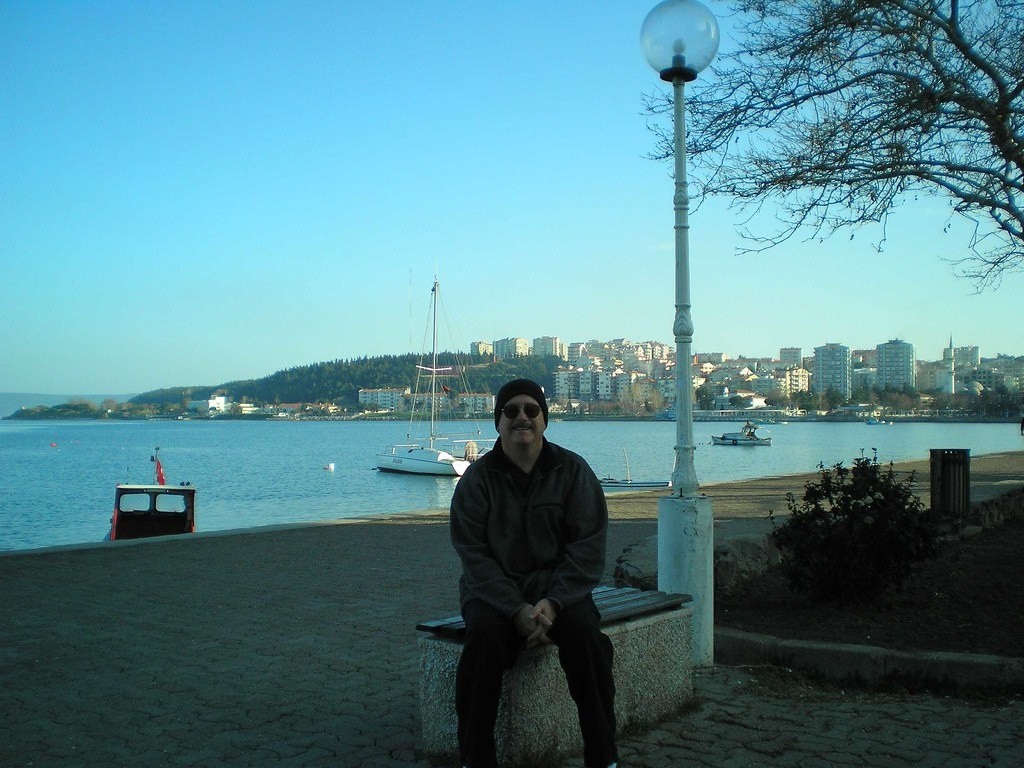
[416,586,693,768]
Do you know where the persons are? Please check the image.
[449,377,622,768]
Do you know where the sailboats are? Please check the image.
[375,252,498,477]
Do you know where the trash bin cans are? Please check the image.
[928,448,972,513]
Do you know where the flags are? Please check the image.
[156,460,165,486]
[443,383,450,391]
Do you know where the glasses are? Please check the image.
[499,403,544,418]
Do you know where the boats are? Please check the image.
[866,418,879,425]
[600,452,669,493]
[710,425,773,446]
[101,448,197,542]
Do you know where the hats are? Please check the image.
[495,378,550,426]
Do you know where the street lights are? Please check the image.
[640,1,720,668]
